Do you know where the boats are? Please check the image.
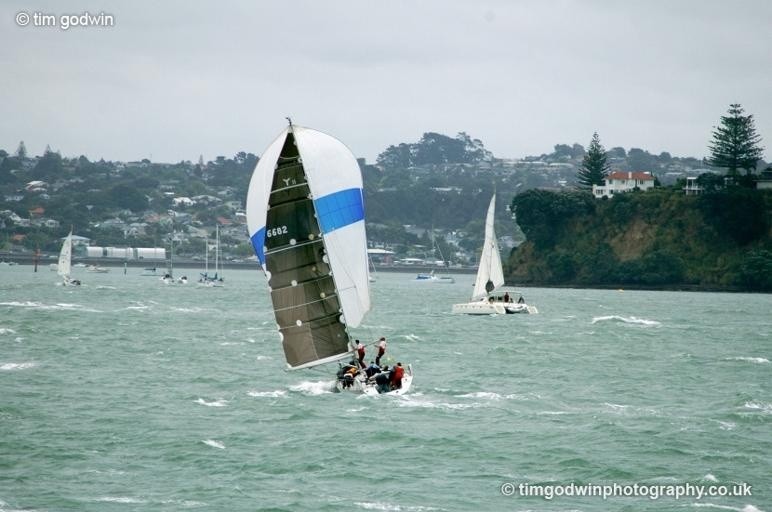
[416,269,455,283]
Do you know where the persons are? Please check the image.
[489,292,525,304]
[351,337,404,390]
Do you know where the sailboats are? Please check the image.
[450,192,539,315]
[53,222,227,291]
[246,114,414,396]
